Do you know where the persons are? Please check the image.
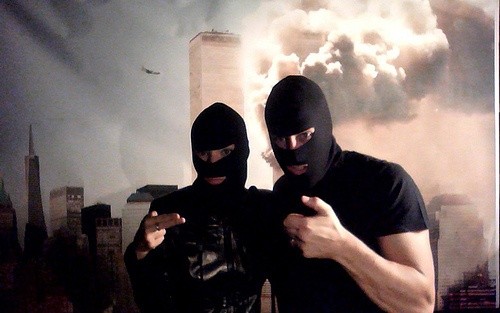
[264,74,436,313]
[122,102,272,313]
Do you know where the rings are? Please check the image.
[155,221,160,230]
[290,238,296,247]
